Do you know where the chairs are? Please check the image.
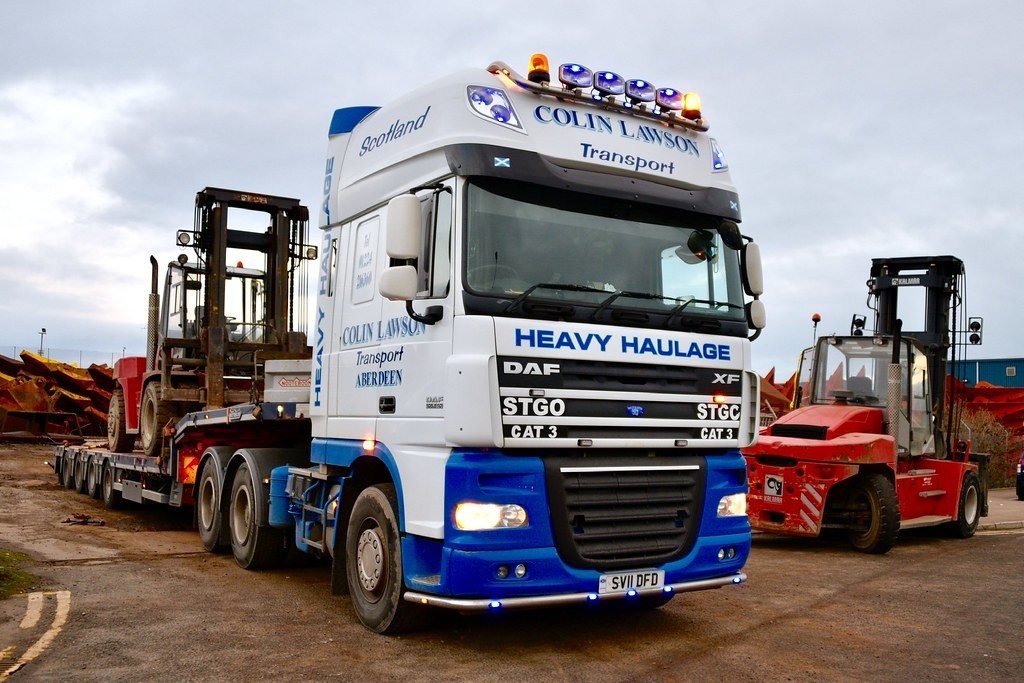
[846,377,872,395]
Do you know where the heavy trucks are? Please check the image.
[45,53,765,635]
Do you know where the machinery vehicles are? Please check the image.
[106,187,318,453]
[744,254,993,554]
